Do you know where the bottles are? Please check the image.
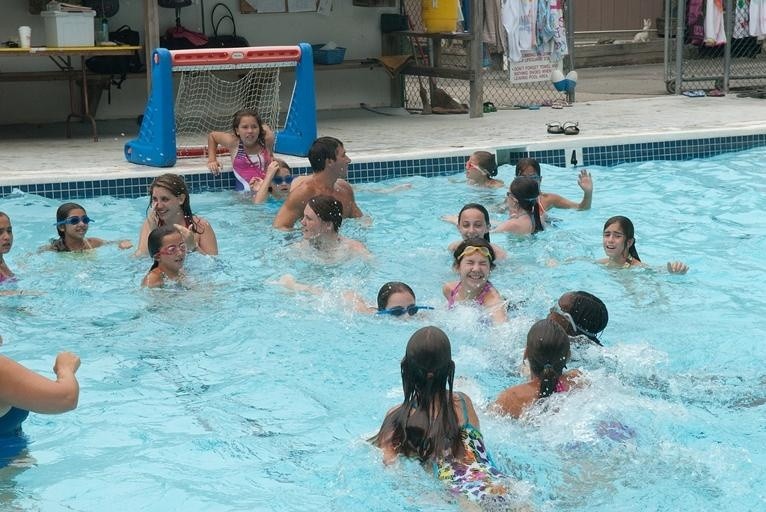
[100,19,109,42]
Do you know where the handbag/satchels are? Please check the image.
[209,3,250,49]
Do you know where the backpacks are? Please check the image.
[86,25,140,74]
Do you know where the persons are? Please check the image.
[24,201,133,263]
[0,210,39,295]
[130,172,217,258]
[365,325,532,512]
[0,337,82,477]
[566,215,690,274]
[140,225,187,289]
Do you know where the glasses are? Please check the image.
[379,303,418,317]
[457,245,493,263]
[57,215,90,226]
[271,175,295,187]
[159,241,187,257]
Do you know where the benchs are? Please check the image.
[0,57,420,110]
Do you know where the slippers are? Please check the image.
[562,120,580,136]
[516,97,574,112]
[683,87,725,98]
[546,120,564,133]
[483,101,498,113]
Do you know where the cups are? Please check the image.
[17,26,31,49]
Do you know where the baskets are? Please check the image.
[312,42,348,63]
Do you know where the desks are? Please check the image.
[0,40,138,141]
